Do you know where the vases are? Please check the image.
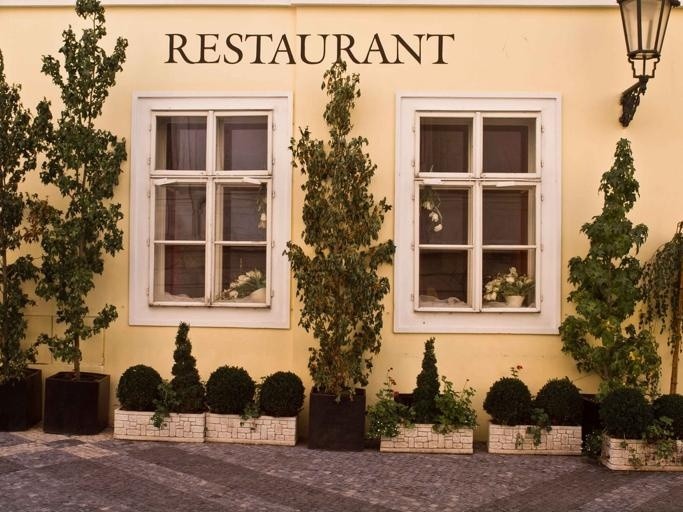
[505,295,525,308]
[248,286,266,304]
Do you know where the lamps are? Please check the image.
[616,0,680,128]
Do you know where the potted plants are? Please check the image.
[112,322,306,445]
[480,375,587,461]
[364,333,483,456]
[598,389,682,472]
[284,59,397,452]
[0,1,126,439]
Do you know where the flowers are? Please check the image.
[222,269,264,300]
[420,185,444,232]
[482,265,535,303]
